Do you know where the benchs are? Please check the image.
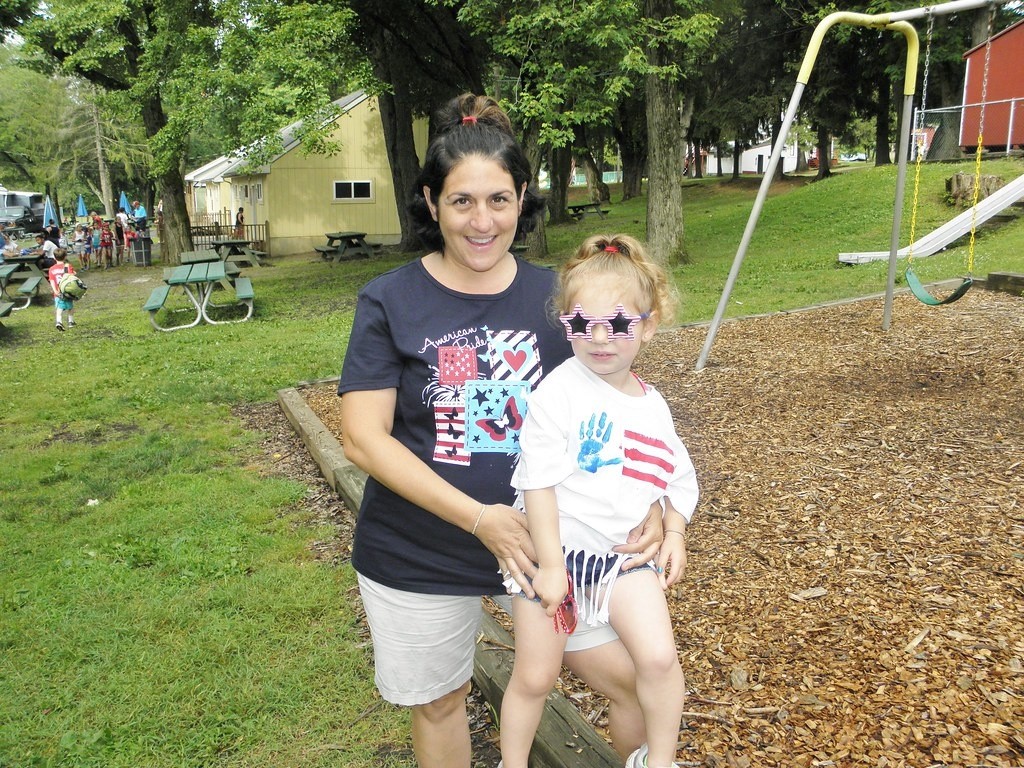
[236,246,268,258]
[314,242,383,260]
[571,209,611,217]
[142,267,187,332]
[0,276,42,322]
[224,262,255,324]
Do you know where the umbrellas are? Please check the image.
[77,194,88,217]
[41,195,60,227]
[120,192,132,215]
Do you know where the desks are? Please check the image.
[0,263,20,302]
[168,261,227,285]
[325,232,378,265]
[211,240,261,269]
[3,252,55,297]
[567,202,606,222]
[180,249,220,265]
[4,227,26,241]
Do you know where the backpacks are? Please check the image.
[57,262,88,301]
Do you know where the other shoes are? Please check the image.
[80,268,84,273]
[126,258,130,262]
[84,264,88,270]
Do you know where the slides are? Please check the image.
[836,173,1024,265]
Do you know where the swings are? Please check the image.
[904,14,994,306]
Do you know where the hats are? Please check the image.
[101,222,110,227]
[133,201,139,207]
[82,226,88,232]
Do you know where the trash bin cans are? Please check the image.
[130,237,154,266]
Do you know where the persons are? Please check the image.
[48,248,78,331]
[29,235,59,282]
[337,91,651,768]
[72,212,136,272]
[117,207,130,231]
[41,219,62,247]
[233,207,246,241]
[495,233,702,768]
[154,200,168,236]
[0,223,22,266]
[131,201,148,235]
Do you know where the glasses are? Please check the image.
[558,303,652,345]
[554,564,579,637]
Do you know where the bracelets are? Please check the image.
[470,504,485,535]
[664,529,685,537]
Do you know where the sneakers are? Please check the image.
[624,742,683,768]
[68,321,78,328]
[55,321,66,333]
